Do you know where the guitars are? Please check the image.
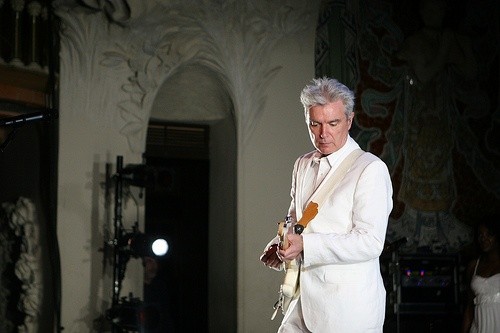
[271,215,302,321]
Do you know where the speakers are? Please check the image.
[394,255,460,333]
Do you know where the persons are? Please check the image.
[142,255,163,333]
[463,220,500,333]
[260,76,393,333]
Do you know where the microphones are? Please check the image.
[2,110,59,126]
